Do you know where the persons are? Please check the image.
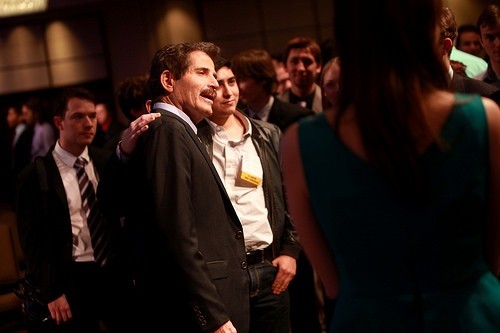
[0,1,499,333]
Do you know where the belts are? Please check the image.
[247,248,272,265]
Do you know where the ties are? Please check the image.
[73,159,107,266]
